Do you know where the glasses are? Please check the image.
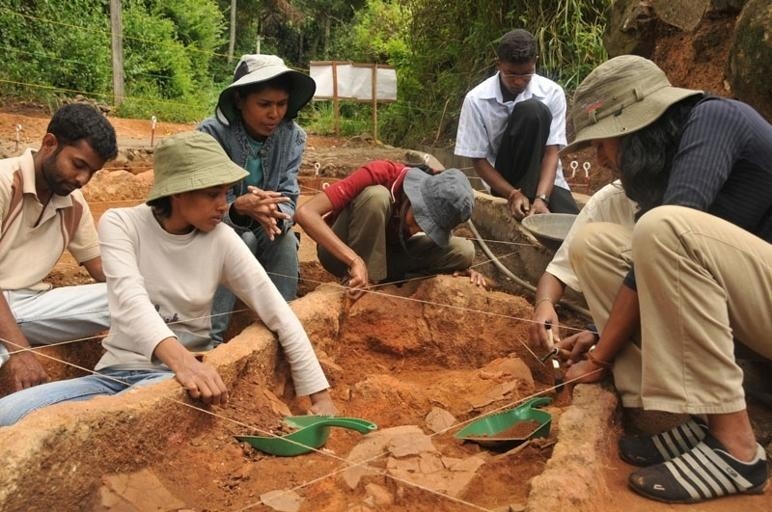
[499,62,536,78]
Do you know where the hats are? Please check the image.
[557,55,705,159]
[146,129,250,206]
[403,167,475,248]
[216,53,316,128]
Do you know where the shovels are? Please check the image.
[515,336,582,370]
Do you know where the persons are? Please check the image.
[0,129,339,424]
[453,30,581,253]
[196,54,317,348]
[0,102,119,391]
[549,54,772,502]
[401,151,441,176]
[293,158,488,301]
[531,179,646,354]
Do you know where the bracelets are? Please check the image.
[348,255,360,267]
[533,297,552,312]
[507,188,521,199]
[587,345,612,369]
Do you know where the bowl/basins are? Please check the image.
[520,211,578,250]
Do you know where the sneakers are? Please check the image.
[627,433,769,504]
[617,419,709,466]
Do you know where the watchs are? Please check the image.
[534,194,550,205]
